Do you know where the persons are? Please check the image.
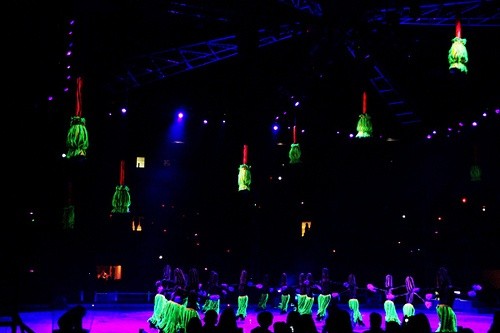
[305,223,309,233]
[54,265,500,333]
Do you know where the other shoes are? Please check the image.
[236,315,239,319]
[146,319,166,332]
[358,320,364,326]
[281,310,286,314]
[321,316,325,319]
[316,315,321,320]
[241,317,245,320]
